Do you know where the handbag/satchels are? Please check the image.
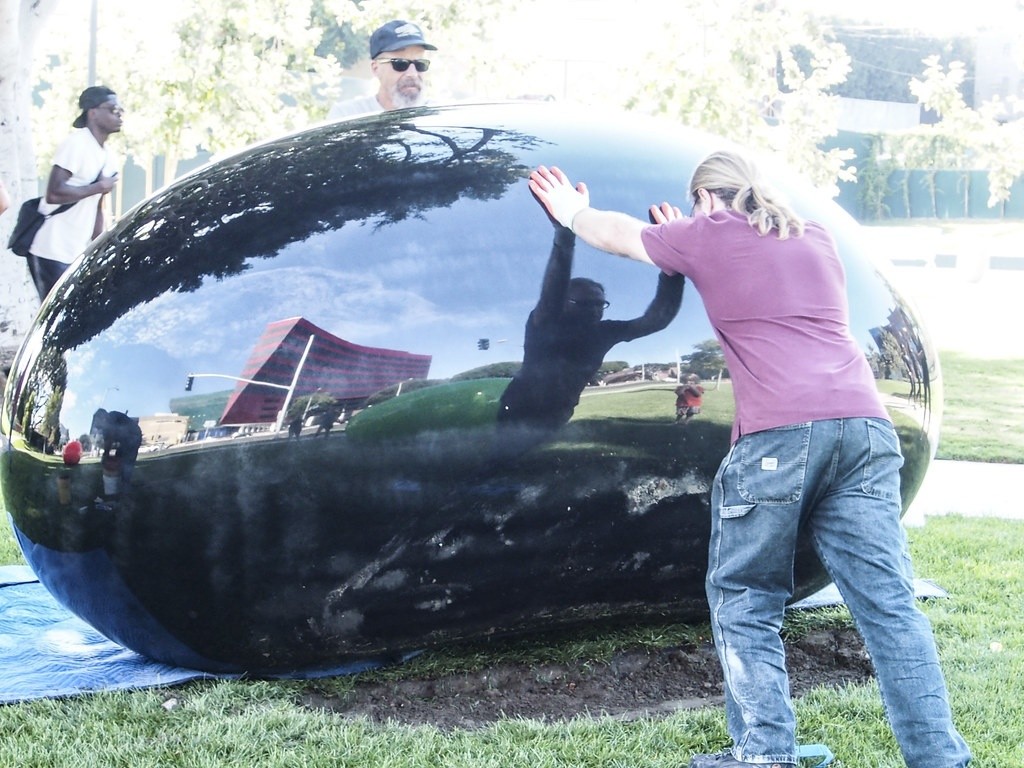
[6,196,46,257]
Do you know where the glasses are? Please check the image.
[95,104,127,115]
[567,298,612,309]
[376,57,430,72]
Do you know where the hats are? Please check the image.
[369,19,438,59]
[71,85,118,129]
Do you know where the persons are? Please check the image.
[528,145,974,768]
[286,416,306,445]
[674,371,705,422]
[494,189,688,428]
[92,409,142,507]
[7,82,123,307]
[314,405,346,441]
[323,21,440,127]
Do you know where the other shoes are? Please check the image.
[689,752,794,767]
